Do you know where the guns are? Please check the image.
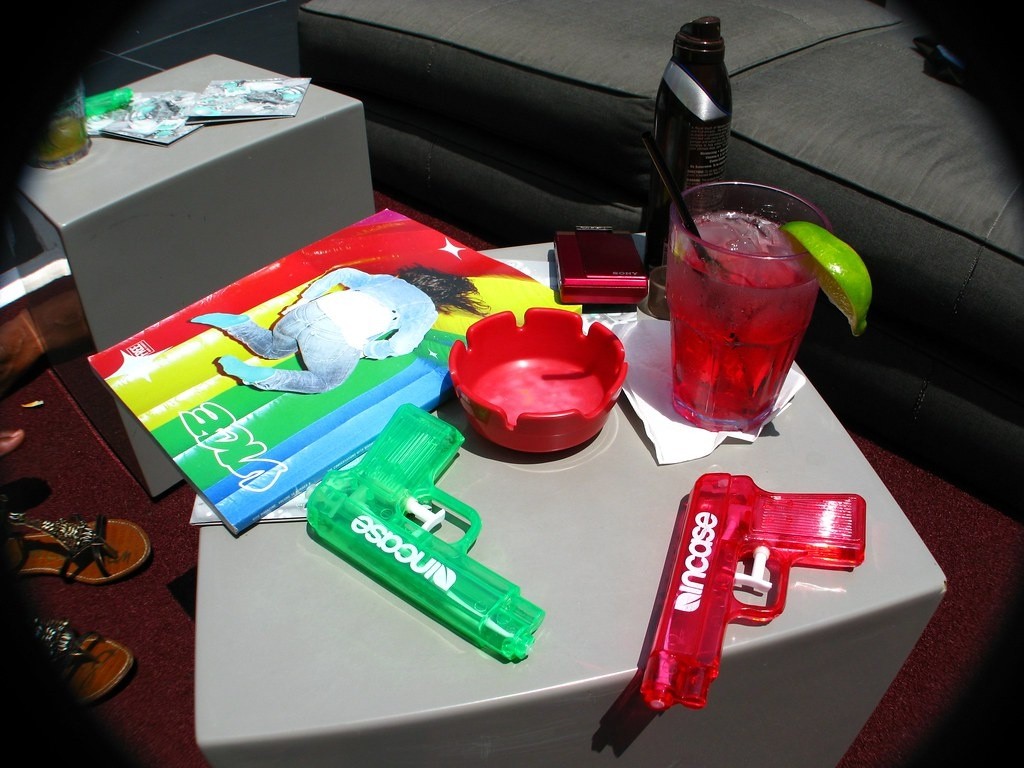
[304,402,546,665]
[639,472,867,713]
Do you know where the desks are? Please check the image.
[14,54,378,502]
[198,308,951,768]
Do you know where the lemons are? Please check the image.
[779,220,873,337]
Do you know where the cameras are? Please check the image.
[554,226,650,306]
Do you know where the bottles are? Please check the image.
[644,18,734,321]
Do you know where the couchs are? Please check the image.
[303,0,1024,522]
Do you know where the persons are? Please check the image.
[189,265,491,394]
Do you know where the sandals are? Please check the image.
[23,617,134,703]
[1,512,152,582]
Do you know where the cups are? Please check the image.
[668,181,832,432]
[35,81,94,169]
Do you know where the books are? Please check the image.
[87,208,586,539]
